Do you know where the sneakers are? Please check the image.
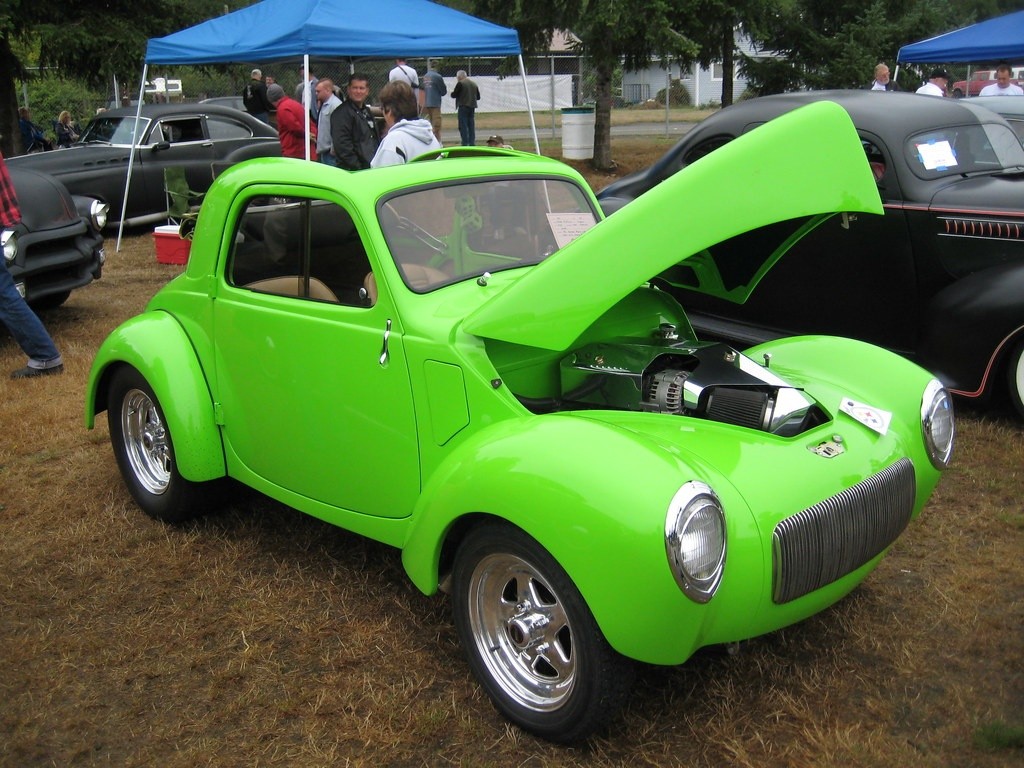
[11,364,64,378]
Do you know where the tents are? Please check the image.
[891,11,1024,97]
[115,0,553,255]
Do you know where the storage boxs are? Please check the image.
[153,225,190,265]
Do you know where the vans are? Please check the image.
[143,79,182,95]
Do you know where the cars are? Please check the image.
[0,167,110,320]
[589,88,1024,426]
[195,96,248,114]
[82,98,955,751]
[0,106,284,232]
[957,93,1024,171]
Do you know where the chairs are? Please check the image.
[246,275,336,305]
[363,261,453,308]
[211,161,234,178]
[181,124,202,139]
[164,164,205,235]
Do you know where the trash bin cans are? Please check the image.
[561,106,596,159]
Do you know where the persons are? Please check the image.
[0,152,65,379]
[16,105,116,153]
[242,56,516,277]
[864,63,1024,154]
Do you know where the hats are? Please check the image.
[930,68,953,79]
[266,84,285,103]
[486,135,504,144]
